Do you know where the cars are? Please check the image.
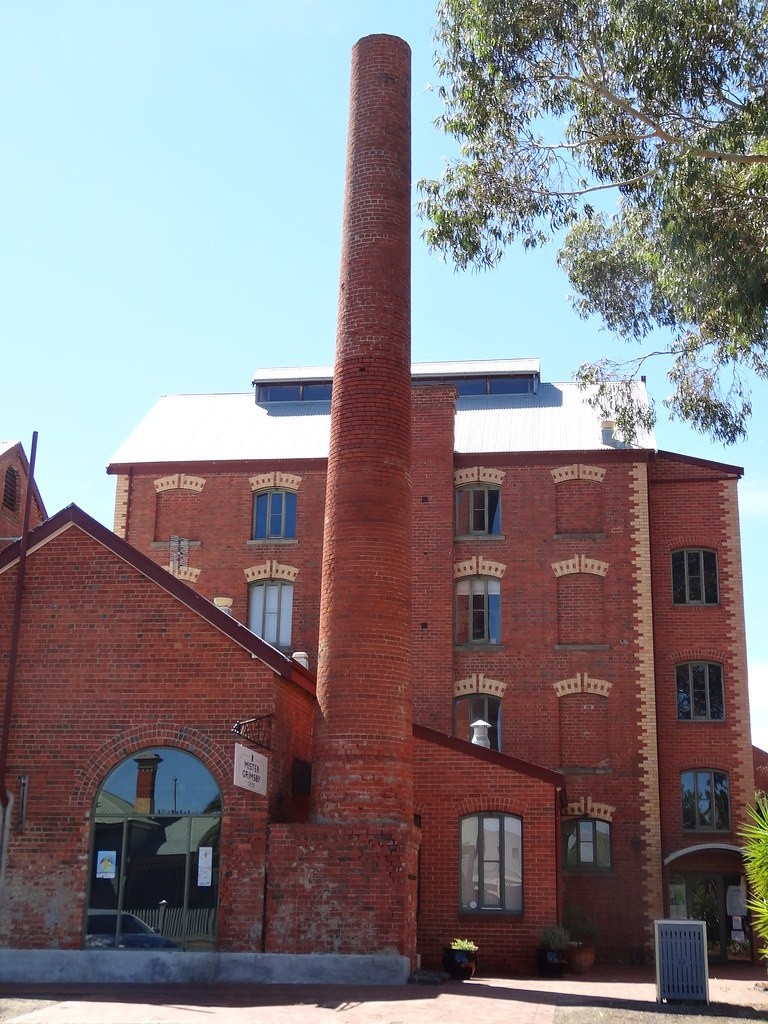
[86,909,179,950]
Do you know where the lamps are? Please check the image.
[470,718,493,749]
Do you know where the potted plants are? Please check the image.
[442,937,480,979]
[535,923,572,977]
[562,901,602,974]
